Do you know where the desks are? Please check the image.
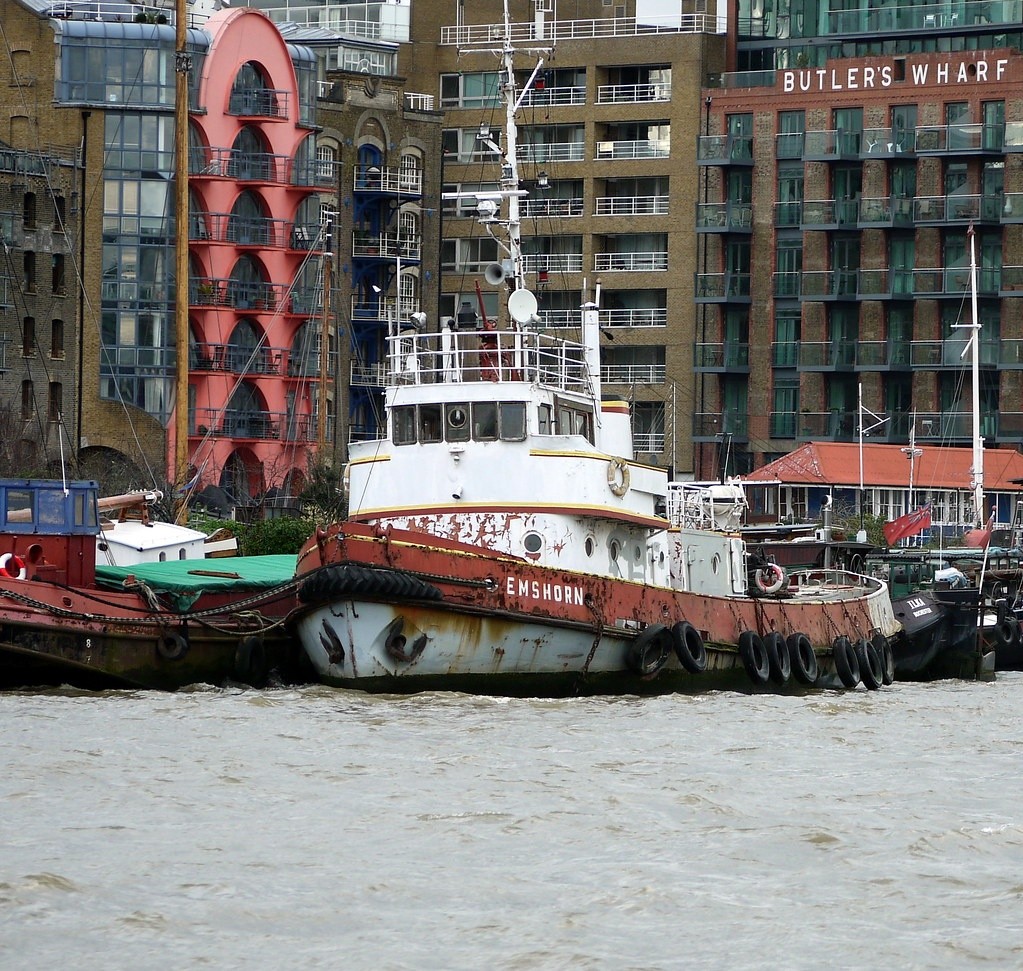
[715,212,728,226]
[712,351,724,368]
[936,13,946,28]
[710,142,725,159]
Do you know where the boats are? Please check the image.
[294,0,900,690]
[743,236,1023,680]
[0,478,299,687]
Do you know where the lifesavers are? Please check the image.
[234,635,264,682]
[785,632,818,685]
[739,630,770,684]
[833,638,861,687]
[993,620,1023,646]
[672,620,707,674]
[855,638,883,689]
[158,632,190,661]
[608,457,631,495]
[755,562,784,593]
[0,552,26,580]
[871,635,895,685]
[626,623,674,677]
[763,631,791,682]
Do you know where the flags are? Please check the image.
[883,503,931,545]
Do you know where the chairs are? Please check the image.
[923,15,936,28]
[703,208,751,228]
[946,14,954,27]
[698,276,725,297]
